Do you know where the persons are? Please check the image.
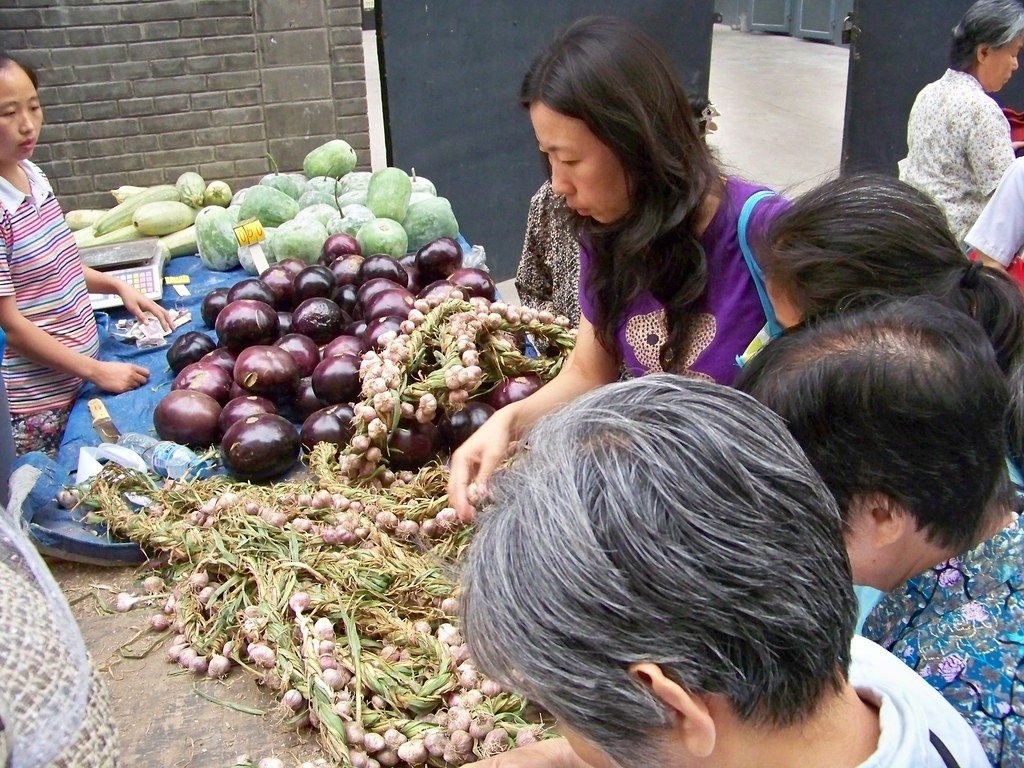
[733,293,1024,768]
[446,15,797,526]
[963,156,1024,290]
[897,0,1024,246]
[0,51,176,464]
[455,373,987,768]
[758,172,1024,480]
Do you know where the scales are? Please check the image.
[78,235,171,311]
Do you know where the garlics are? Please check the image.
[57,290,582,768]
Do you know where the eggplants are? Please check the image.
[153,233,549,486]
